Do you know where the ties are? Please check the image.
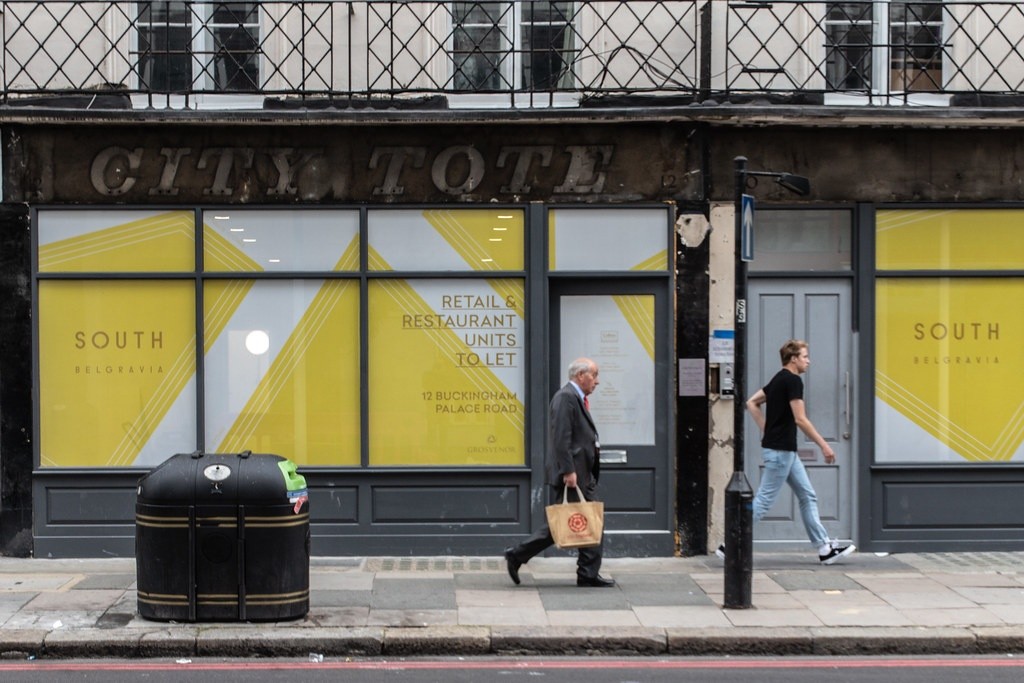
[584,396,589,411]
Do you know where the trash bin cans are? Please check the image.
[134,451,311,623]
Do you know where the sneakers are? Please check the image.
[818,539,856,565]
[716,543,725,559]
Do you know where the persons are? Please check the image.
[504,356,617,588]
[715,340,856,564]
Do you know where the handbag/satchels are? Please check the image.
[545,483,604,550]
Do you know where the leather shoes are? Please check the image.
[506,547,520,585]
[576,573,616,588]
